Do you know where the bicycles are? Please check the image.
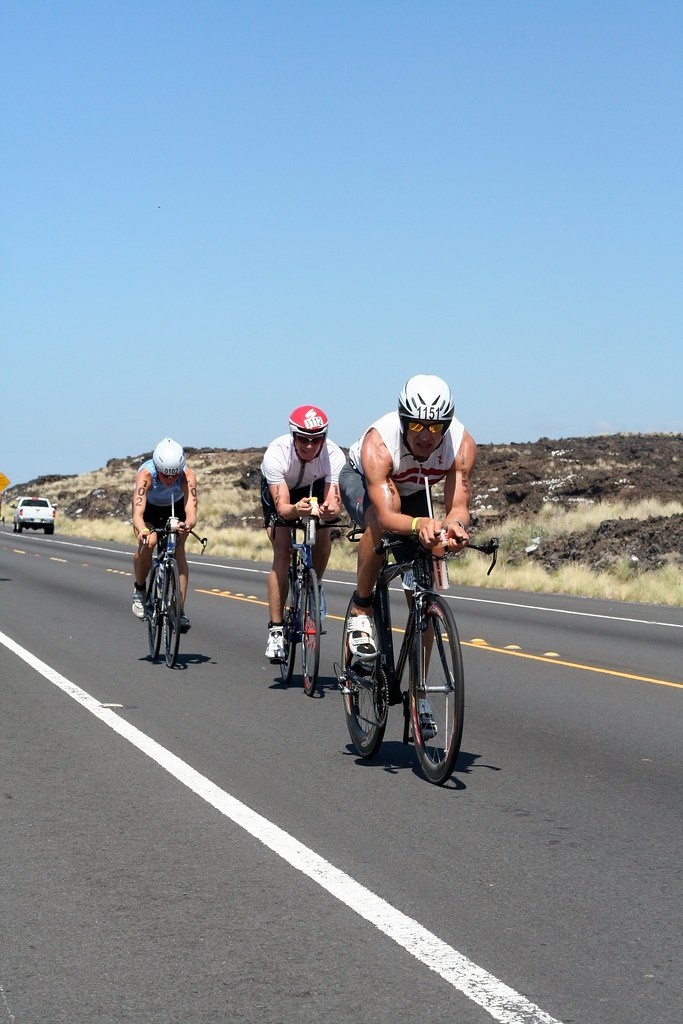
[332,477,501,785]
[268,475,345,699]
[139,493,208,669]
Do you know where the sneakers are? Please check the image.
[418,698,437,742]
[131,589,147,619]
[264,625,285,658]
[177,614,190,634]
[309,584,326,622]
[347,610,380,661]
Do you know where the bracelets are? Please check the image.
[446,519,465,530]
[295,502,299,512]
[412,517,420,535]
[140,528,149,533]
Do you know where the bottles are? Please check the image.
[295,576,303,596]
[167,516,179,554]
[157,563,171,599]
[306,496,317,545]
[431,530,449,591]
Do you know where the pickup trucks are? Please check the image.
[9,497,56,535]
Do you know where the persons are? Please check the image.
[132,437,197,631]
[338,374,477,737]
[260,405,346,659]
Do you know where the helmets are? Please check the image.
[289,406,329,440]
[398,375,454,434]
[153,437,186,475]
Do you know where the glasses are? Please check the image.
[159,469,182,478]
[401,416,449,433]
[294,433,326,444]
